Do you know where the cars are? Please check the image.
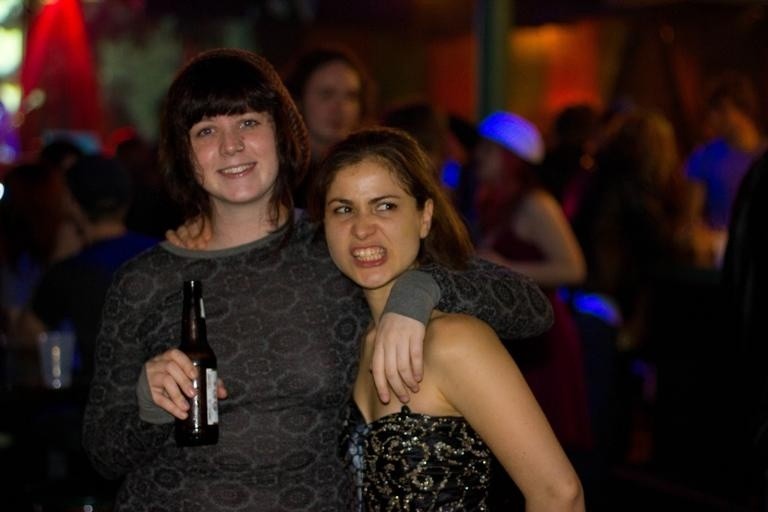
[38,329,78,389]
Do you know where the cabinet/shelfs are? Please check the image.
[175,280,220,445]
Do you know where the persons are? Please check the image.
[165,125,586,512]
[81,47,557,511]
[285,39,768,477]
[2,123,214,477]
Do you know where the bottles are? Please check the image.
[475,110,548,165]
